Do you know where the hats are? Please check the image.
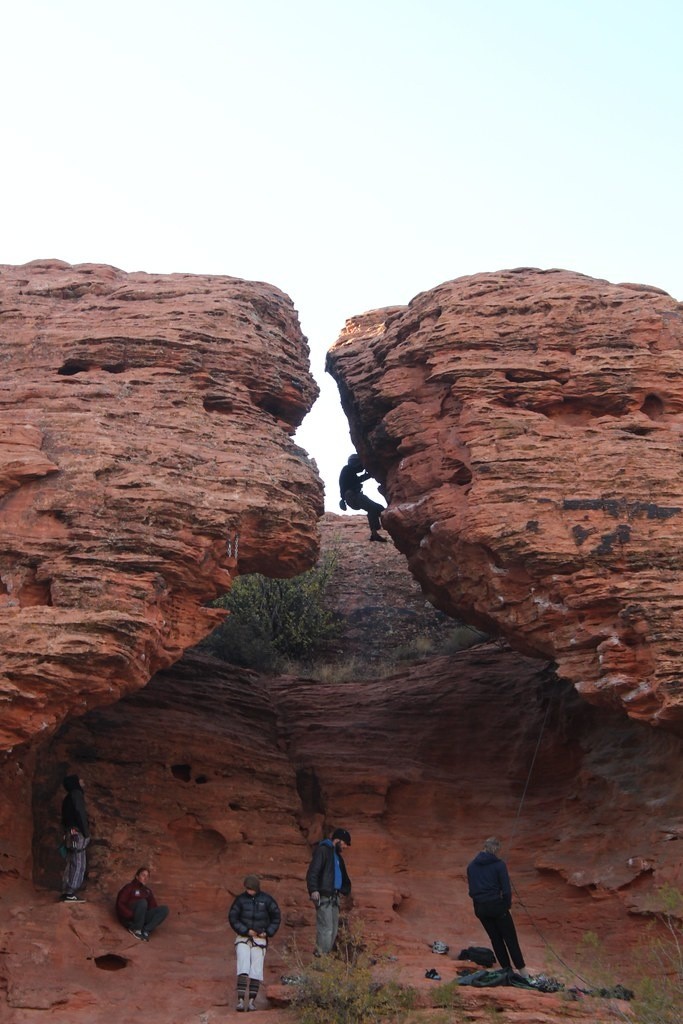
[244,876,260,890]
[332,829,351,846]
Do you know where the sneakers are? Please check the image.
[63,894,85,903]
[128,928,149,943]
[236,1002,257,1011]
[432,940,449,954]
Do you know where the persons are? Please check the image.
[62,774,91,902]
[339,454,387,542]
[467,836,541,979]
[228,876,281,1011]
[117,867,169,942]
[306,828,352,957]
